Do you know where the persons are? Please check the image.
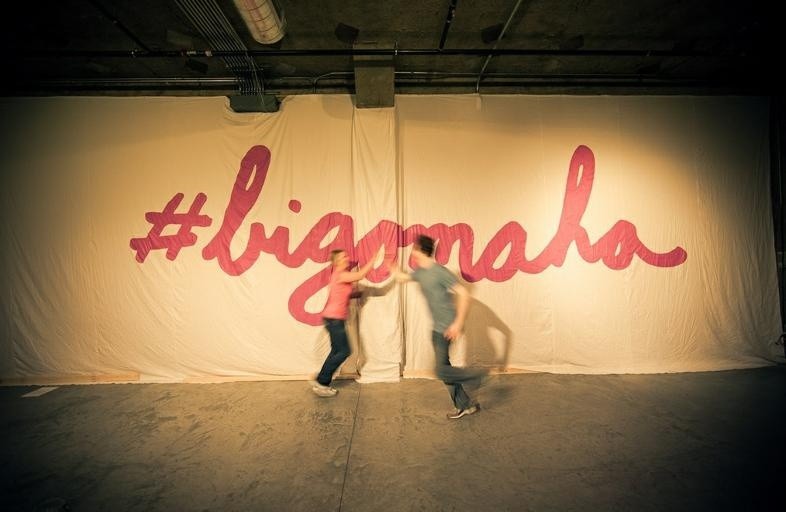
[313,249,377,397]
[385,234,489,420]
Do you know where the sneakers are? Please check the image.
[313,380,339,398]
[446,404,477,420]
